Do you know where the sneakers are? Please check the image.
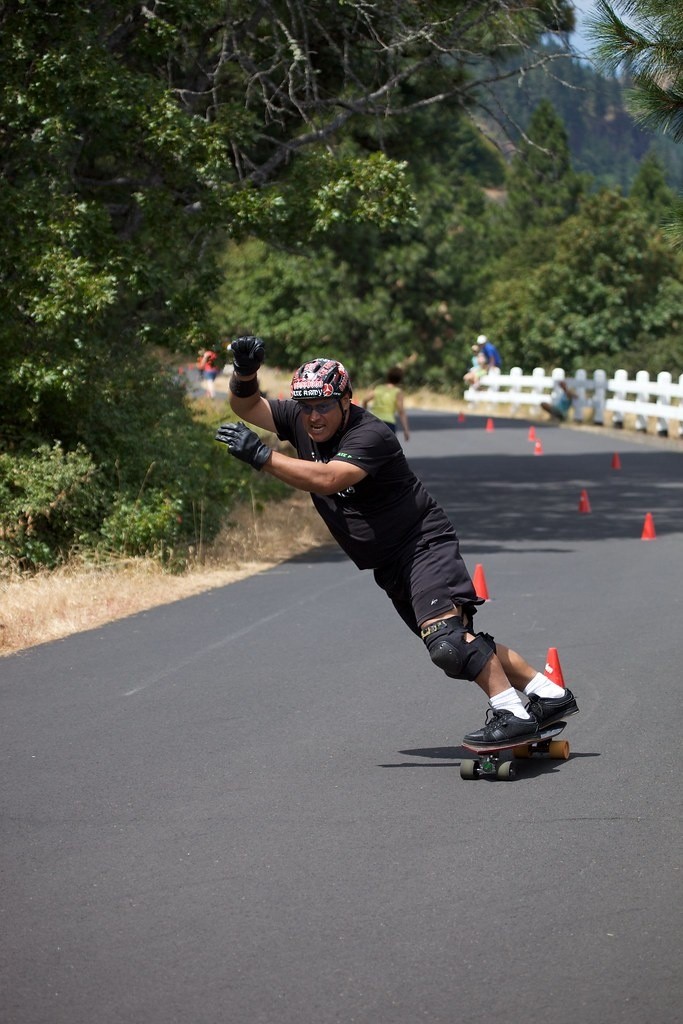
[524,687,579,729]
[460,710,541,751]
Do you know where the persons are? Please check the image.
[215,337,580,747]
[541,382,578,422]
[463,335,501,391]
[362,368,409,440]
[198,347,219,399]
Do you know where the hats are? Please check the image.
[477,334,487,345]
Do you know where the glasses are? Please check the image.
[299,400,337,414]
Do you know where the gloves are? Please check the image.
[231,336,266,375]
[215,421,272,472]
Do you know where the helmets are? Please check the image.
[290,358,352,400]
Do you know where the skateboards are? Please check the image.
[460,721,570,791]
